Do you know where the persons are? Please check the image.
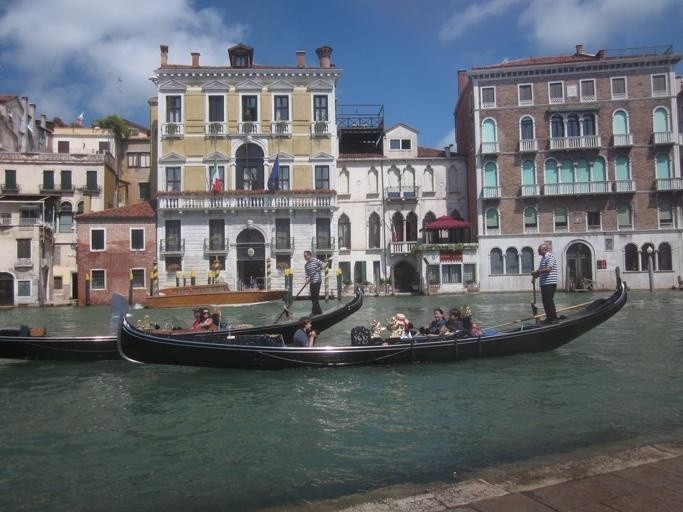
[192,304,218,331]
[293,317,315,347]
[531,243,559,326]
[304,250,326,317]
[389,304,474,340]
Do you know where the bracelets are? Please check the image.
[536,270,539,273]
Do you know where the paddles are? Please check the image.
[530,275,541,322]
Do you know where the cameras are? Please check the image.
[314,328,321,335]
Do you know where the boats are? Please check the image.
[0,282,367,364]
[139,283,290,310]
[107,266,631,372]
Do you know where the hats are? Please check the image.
[192,306,202,312]
[392,313,410,325]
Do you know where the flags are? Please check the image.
[268,154,279,192]
[210,159,222,192]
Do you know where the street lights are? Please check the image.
[635,245,661,292]
